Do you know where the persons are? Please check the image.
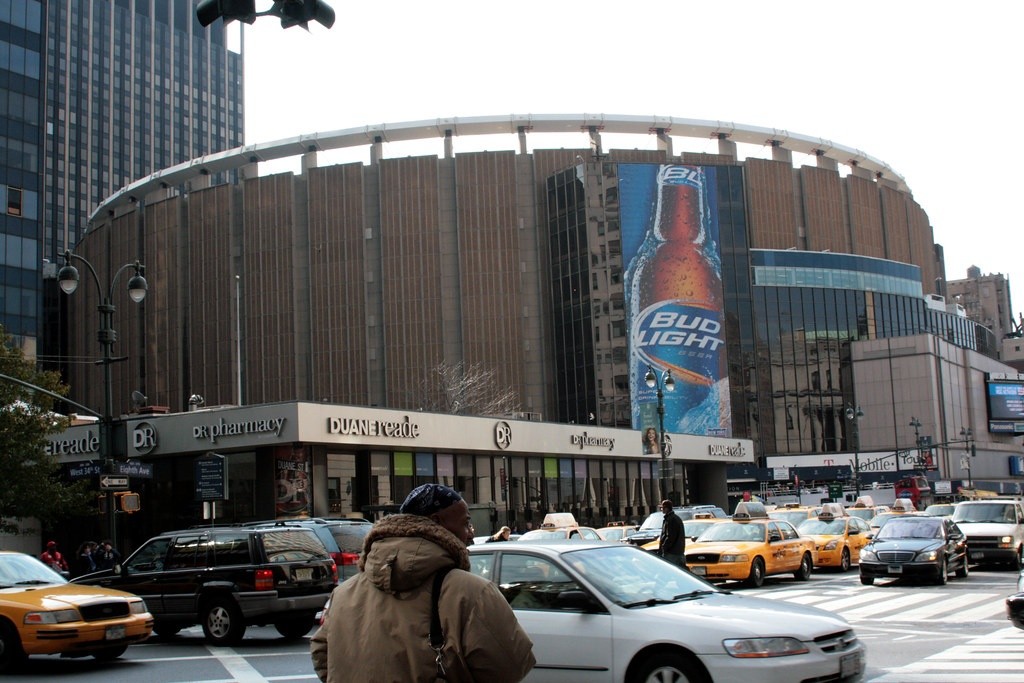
[657,500,686,568]
[642,427,660,454]
[39,540,69,573]
[309,483,537,683]
[76,540,122,576]
[484,519,543,543]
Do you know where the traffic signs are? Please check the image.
[99,474,130,491]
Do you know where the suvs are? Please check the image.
[234,517,377,621]
[65,521,339,649]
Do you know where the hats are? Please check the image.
[401,484,462,517]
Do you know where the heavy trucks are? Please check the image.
[894,474,1024,517]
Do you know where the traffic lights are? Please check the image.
[121,494,141,513]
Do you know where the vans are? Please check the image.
[949,498,1024,571]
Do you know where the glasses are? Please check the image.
[660,507,666,510]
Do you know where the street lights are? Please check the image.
[55,247,150,568]
[959,426,977,490]
[907,414,924,473]
[644,364,676,499]
[845,401,865,501]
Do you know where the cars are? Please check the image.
[844,495,891,522]
[796,502,878,573]
[857,515,970,586]
[924,503,957,517]
[764,502,822,529]
[0,551,155,673]
[1006,591,1024,630]
[678,502,816,589]
[463,538,868,683]
[869,498,921,536]
[472,505,734,555]
